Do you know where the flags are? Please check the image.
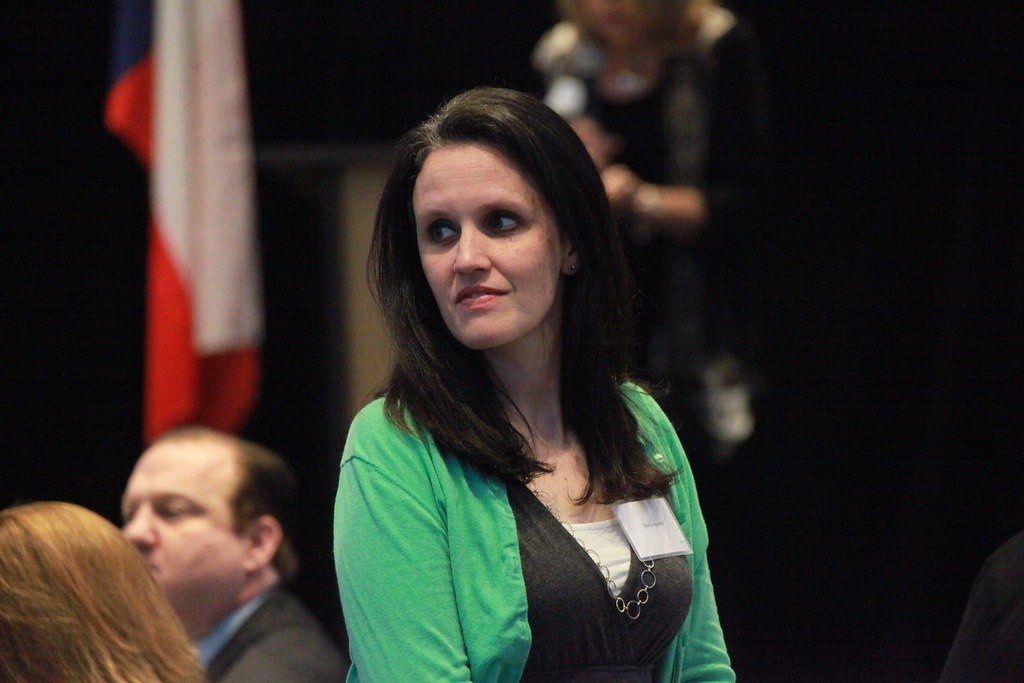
[105,0,264,444]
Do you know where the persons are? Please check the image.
[118,427,348,683]
[330,87,738,683]
[522,0,768,489]
[0,501,207,683]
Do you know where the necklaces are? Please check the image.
[525,478,656,619]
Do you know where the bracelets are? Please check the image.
[625,177,640,215]
